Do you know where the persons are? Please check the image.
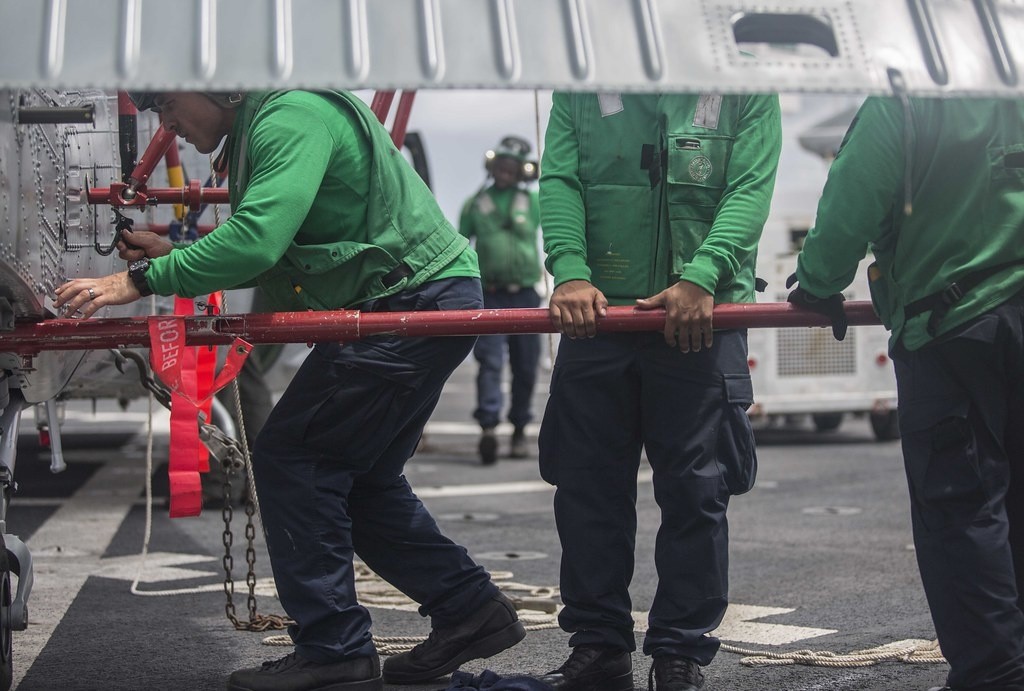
[457,137,540,463]
[788,91,1024,691]
[535,86,784,691]
[54,89,526,691]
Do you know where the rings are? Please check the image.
[87,287,95,299]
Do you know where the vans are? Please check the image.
[731,127,900,442]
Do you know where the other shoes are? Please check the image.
[479,434,498,466]
[510,434,526,458]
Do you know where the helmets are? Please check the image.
[125,91,247,111]
[483,137,539,183]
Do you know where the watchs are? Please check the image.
[128,256,154,296]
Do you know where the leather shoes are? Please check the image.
[649,654,705,691]
[229,645,382,691]
[382,587,527,685]
[533,642,633,691]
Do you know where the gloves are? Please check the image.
[785,272,847,341]
